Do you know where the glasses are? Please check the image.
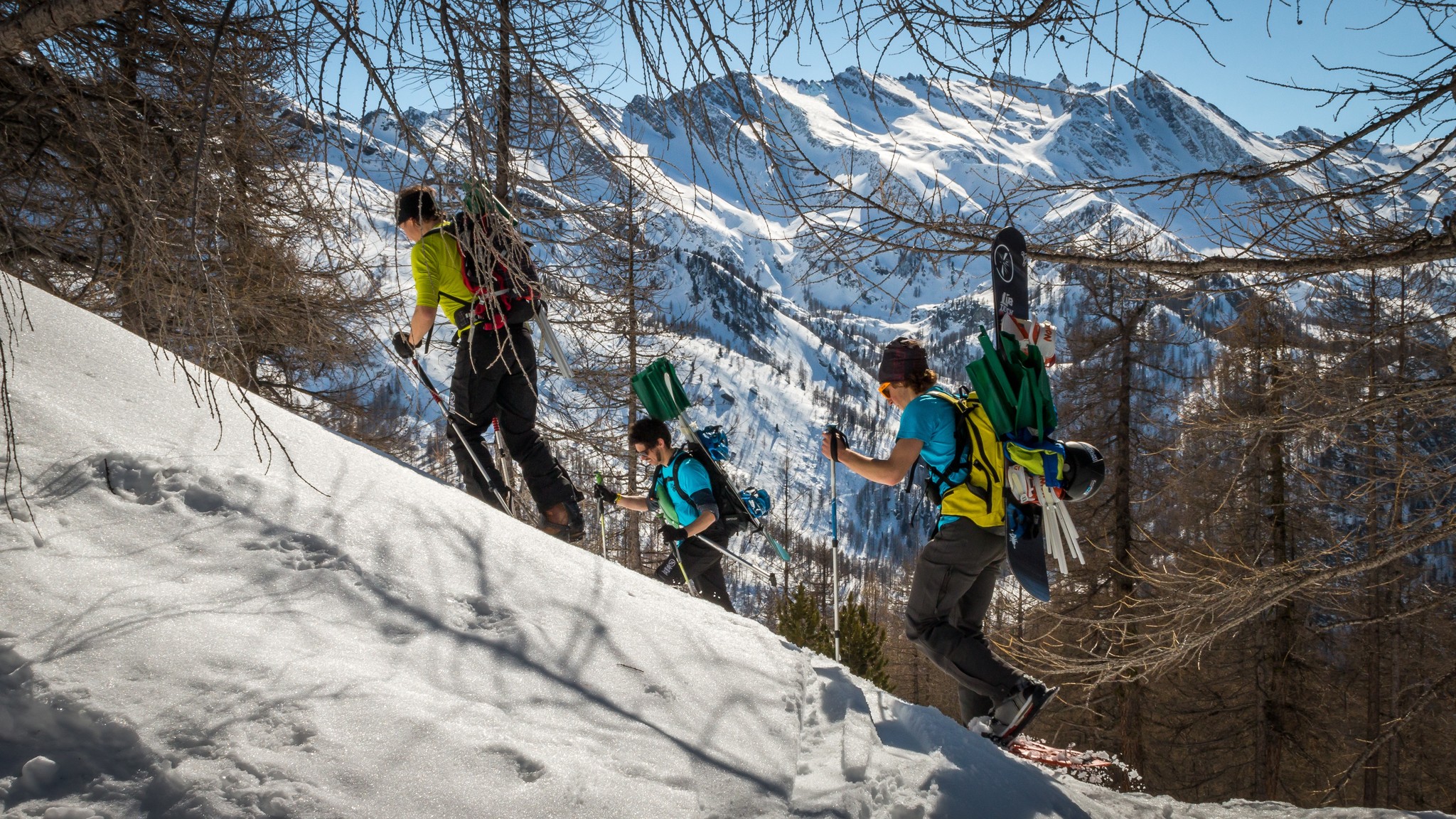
[878,382,891,399]
[636,448,649,457]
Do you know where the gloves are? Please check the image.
[594,483,617,505]
[392,333,422,359]
[658,525,688,543]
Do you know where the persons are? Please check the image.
[592,417,739,614]
[822,337,1061,746]
[392,184,584,543]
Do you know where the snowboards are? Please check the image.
[990,224,1050,603]
[660,372,792,562]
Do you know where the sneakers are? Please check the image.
[535,500,585,544]
[990,676,1045,738]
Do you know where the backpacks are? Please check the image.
[691,455,747,538]
[449,213,535,331]
[958,391,1006,529]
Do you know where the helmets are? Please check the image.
[1061,441,1105,502]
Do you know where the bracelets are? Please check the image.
[616,493,621,503]
